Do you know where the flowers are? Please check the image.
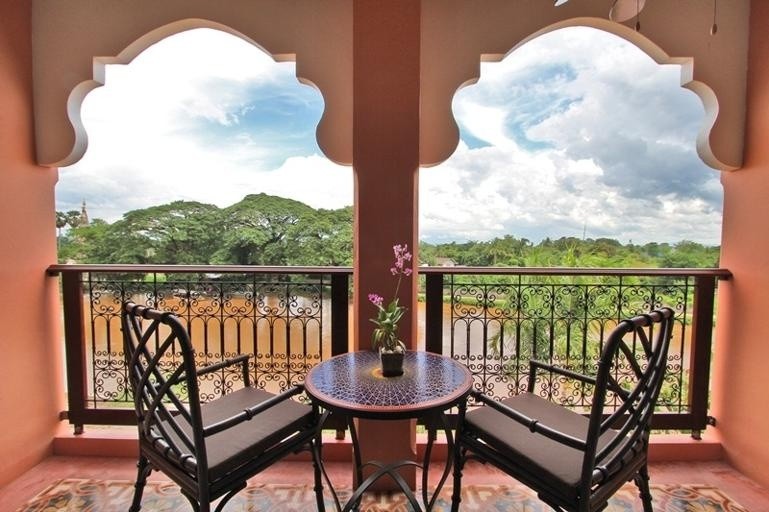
[368,244,413,351]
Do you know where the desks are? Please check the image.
[305,350,474,512]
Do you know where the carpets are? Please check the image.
[15,478,749,512]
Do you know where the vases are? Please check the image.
[379,349,404,377]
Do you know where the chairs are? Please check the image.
[457,307,675,512]
[122,299,327,512]
